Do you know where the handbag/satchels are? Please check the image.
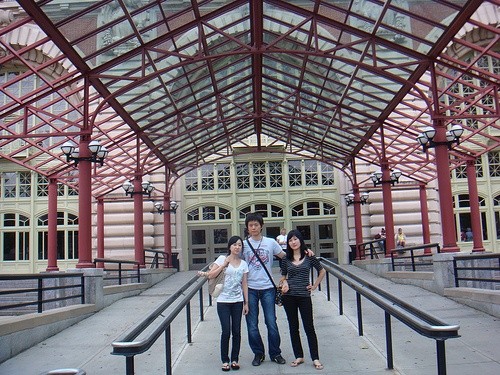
[275,285,283,306]
[208,256,228,298]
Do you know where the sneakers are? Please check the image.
[270,355,285,364]
[252,354,265,365]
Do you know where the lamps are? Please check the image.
[416,124,464,152]
[121,180,154,198]
[60,141,109,166]
[345,192,369,206]
[371,169,401,187]
[154,201,179,214]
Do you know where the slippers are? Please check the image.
[291,359,304,366]
[221,363,230,371]
[231,362,239,369]
[313,362,324,370]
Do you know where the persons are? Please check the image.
[379,228,385,250]
[239,215,314,366]
[460,228,472,241]
[276,228,287,271]
[281,229,326,368]
[395,228,406,253]
[209,235,250,371]
